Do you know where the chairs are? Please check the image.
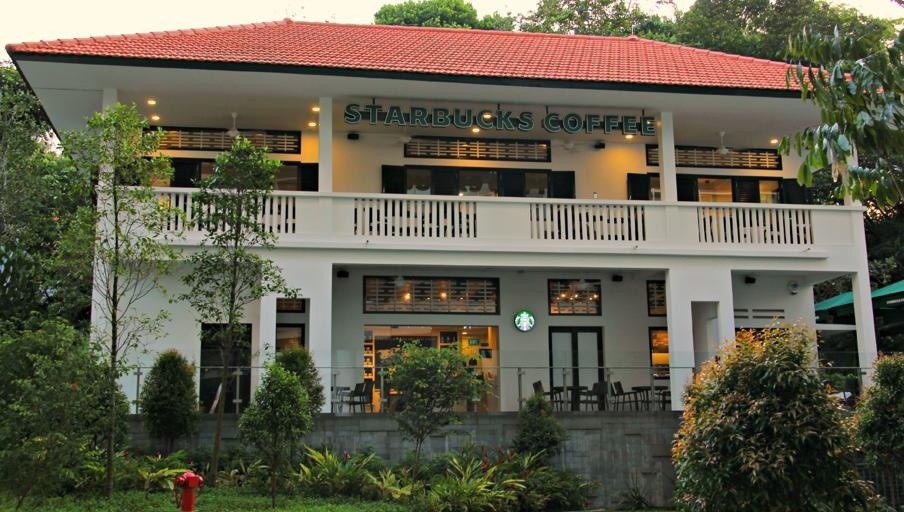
[532,380,671,414]
[341,376,376,413]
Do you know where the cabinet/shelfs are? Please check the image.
[364,327,375,406]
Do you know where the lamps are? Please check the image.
[717,132,728,156]
[563,136,574,150]
[227,112,240,137]
[400,128,411,143]
